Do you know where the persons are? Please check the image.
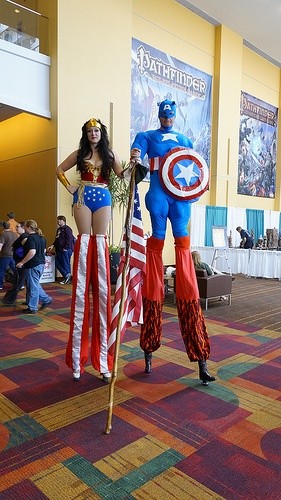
[191,251,235,301]
[0,222,25,291]
[16,220,52,314]
[130,99,199,239]
[236,226,254,249]
[6,211,20,238]
[2,222,29,305]
[56,118,140,235]
[47,215,73,284]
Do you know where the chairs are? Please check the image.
[171,269,232,311]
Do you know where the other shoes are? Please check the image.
[60,276,72,285]
[101,373,111,382]
[145,362,152,373]
[200,371,218,382]
[23,309,38,314]
[72,372,80,381]
[42,301,53,308]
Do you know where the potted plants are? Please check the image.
[108,244,120,266]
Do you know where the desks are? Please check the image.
[39,253,55,284]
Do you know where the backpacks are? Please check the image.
[65,226,75,251]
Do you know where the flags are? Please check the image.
[107,182,146,356]
